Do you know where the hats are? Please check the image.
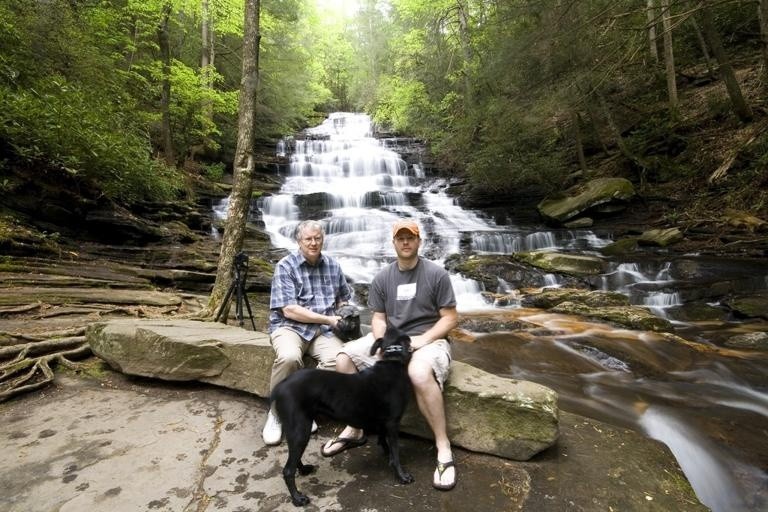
[392,219,420,239]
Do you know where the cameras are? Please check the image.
[232,252,248,265]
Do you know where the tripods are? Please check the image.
[215,270,258,333]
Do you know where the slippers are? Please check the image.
[432,452,456,490]
[320,434,369,458]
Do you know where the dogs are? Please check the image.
[268,328,416,507]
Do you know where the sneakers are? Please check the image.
[261,412,282,446]
[309,421,318,434]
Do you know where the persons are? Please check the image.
[261,220,363,447]
[329,221,459,490]
